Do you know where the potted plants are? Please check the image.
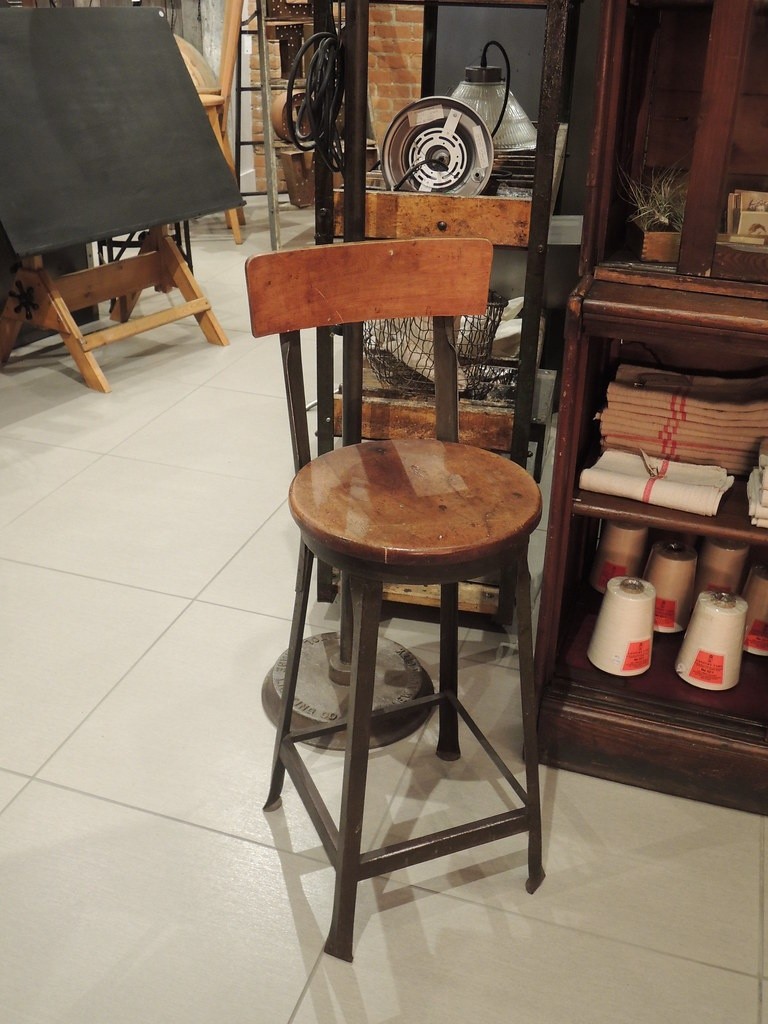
[613,145,683,264]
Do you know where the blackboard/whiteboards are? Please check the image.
[0,4,249,258]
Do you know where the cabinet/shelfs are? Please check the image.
[307,0,580,635]
[521,1,768,820]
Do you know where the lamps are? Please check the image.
[450,40,538,153]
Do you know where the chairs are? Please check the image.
[242,237,547,965]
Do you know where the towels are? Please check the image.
[576,361,768,528]
